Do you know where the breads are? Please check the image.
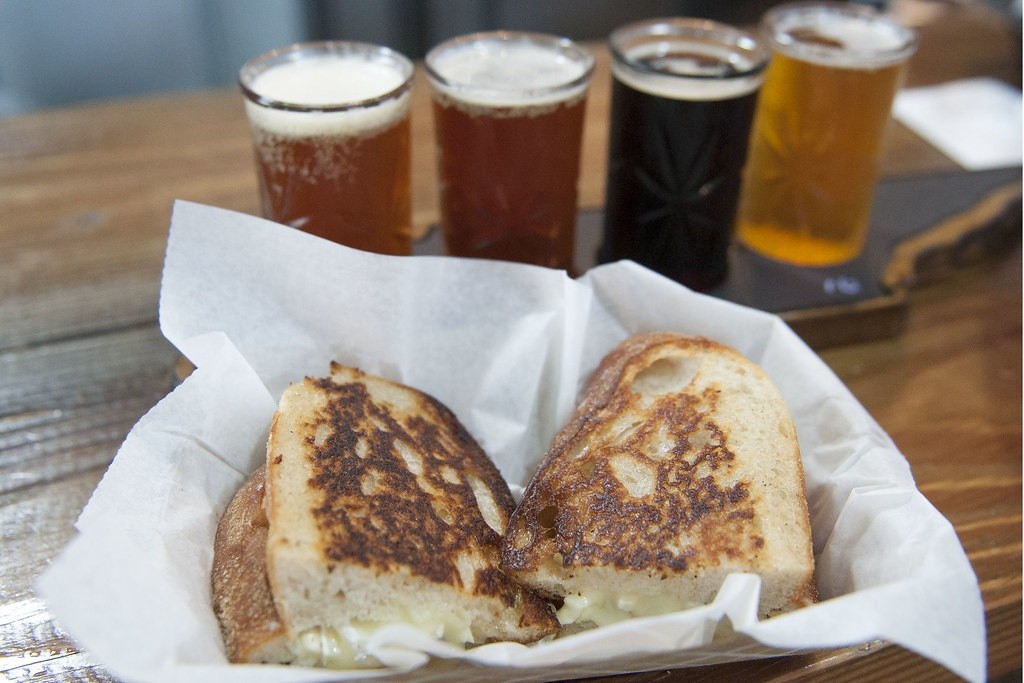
[212,361,563,669]
[497,329,820,628]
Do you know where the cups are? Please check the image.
[235,41,414,258]
[596,15,772,296]
[733,0,922,270]
[422,30,597,278]
